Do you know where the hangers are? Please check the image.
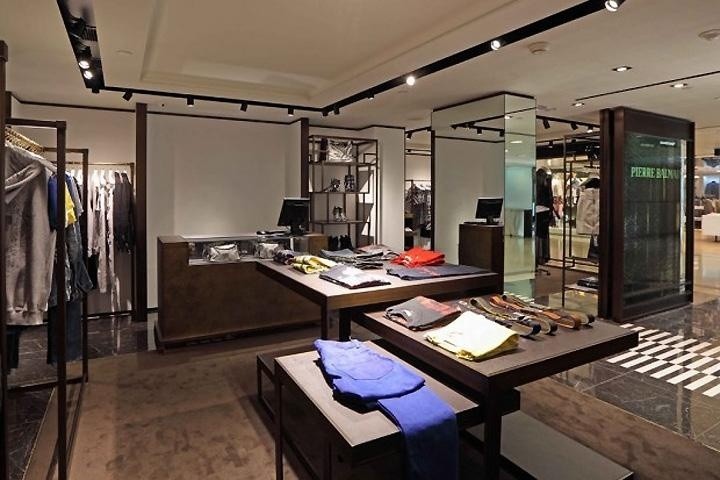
[67,162,130,185]
[4,127,58,169]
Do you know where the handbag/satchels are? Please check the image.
[210,244,240,263]
[320,138,354,161]
[257,243,284,259]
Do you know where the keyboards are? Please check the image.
[463,221,485,225]
[266,230,286,235]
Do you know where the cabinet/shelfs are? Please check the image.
[308,134,378,249]
[562,131,602,308]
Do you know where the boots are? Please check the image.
[333,205,346,221]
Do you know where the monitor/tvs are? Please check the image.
[475,198,503,225]
[277,197,310,237]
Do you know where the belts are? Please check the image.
[469,294,595,337]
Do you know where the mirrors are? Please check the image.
[431,93,536,281]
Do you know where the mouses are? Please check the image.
[256,230,265,235]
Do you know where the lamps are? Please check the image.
[543,119,595,133]
[122,91,295,119]
[70,16,101,95]
[450,124,504,137]
[334,92,375,116]
[406,133,412,141]
